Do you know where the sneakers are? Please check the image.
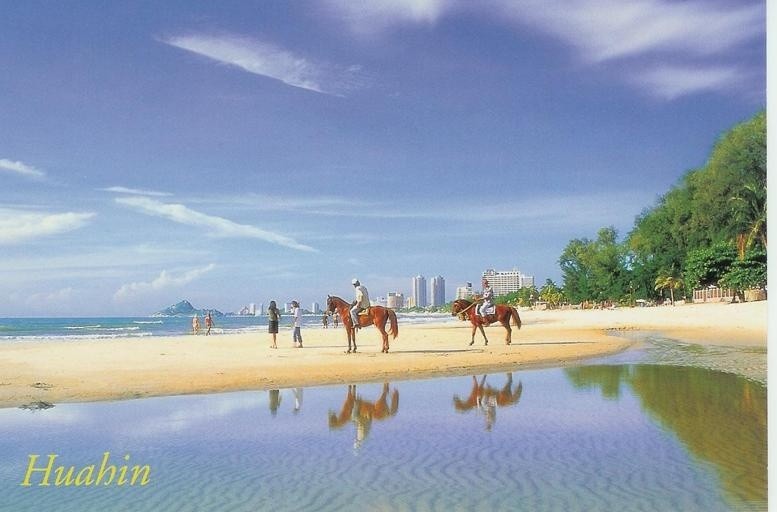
[350,324,360,328]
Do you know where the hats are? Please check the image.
[351,277,360,285]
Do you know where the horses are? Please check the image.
[328,381,400,430]
[326,295,399,355]
[452,299,522,346]
[453,372,523,412]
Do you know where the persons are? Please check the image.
[350,401,372,450]
[291,388,304,414]
[477,389,496,431]
[290,300,304,348]
[205,313,215,336]
[267,301,282,348]
[332,313,338,328]
[269,389,281,418]
[477,279,493,325]
[322,313,328,329]
[192,313,200,336]
[349,278,370,329]
